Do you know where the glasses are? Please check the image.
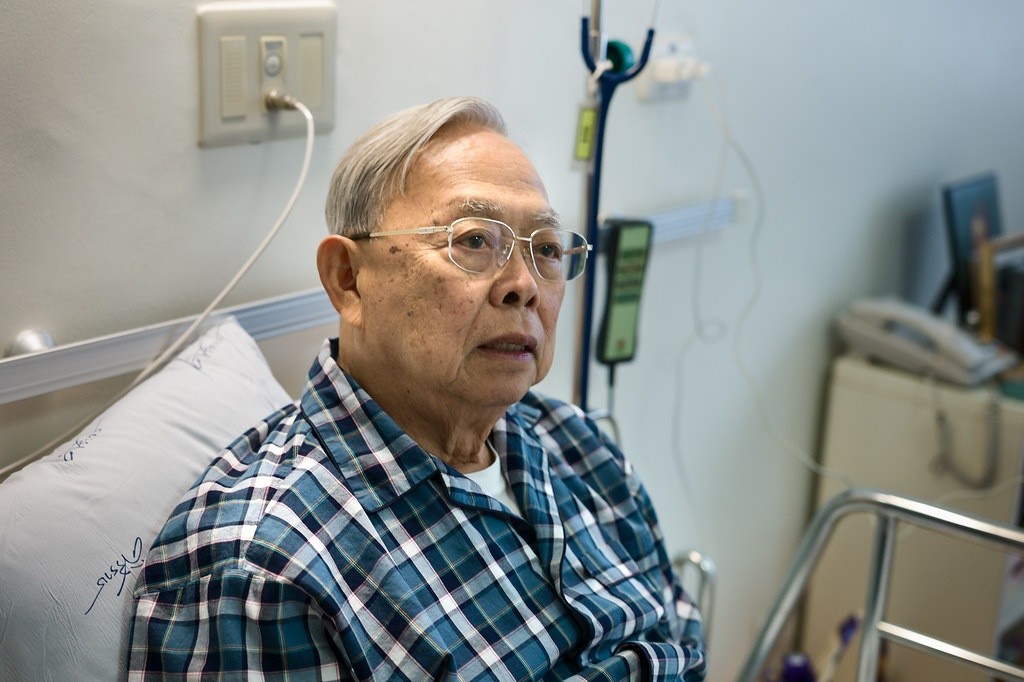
[352,217,594,283]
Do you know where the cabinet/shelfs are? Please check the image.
[797,349,1023,682]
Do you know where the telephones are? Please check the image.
[834,294,1019,387]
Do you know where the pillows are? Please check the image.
[0,316,295,682]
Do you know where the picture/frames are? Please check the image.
[978,230,1023,341]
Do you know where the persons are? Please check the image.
[127,97,709,682]
